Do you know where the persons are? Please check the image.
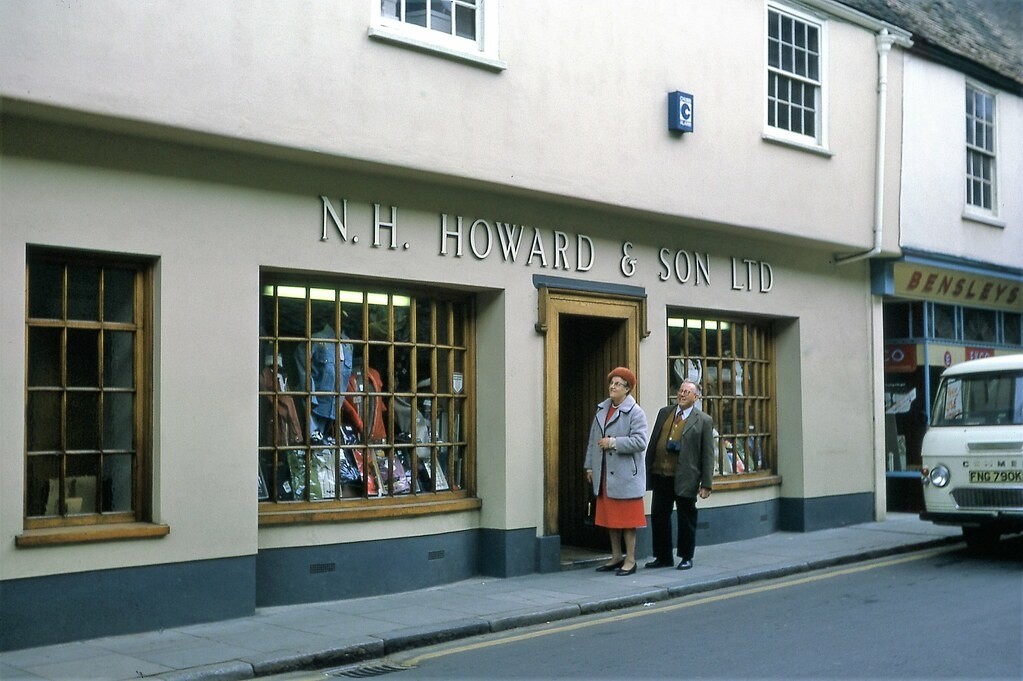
[259,354,303,446]
[295,309,354,436]
[340,356,386,439]
[387,397,432,468]
[645,378,715,570]
[584,367,648,576]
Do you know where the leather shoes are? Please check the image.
[677,559,692,569]
[645,557,674,568]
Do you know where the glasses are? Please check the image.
[678,389,695,396]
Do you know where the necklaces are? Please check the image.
[679,359,696,370]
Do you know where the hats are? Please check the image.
[607,367,635,391]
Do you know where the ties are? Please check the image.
[676,411,682,424]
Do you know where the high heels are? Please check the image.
[616,563,637,576]
[596,559,624,571]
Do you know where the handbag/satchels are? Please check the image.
[586,483,596,519]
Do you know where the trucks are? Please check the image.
[917,354,1023,556]
